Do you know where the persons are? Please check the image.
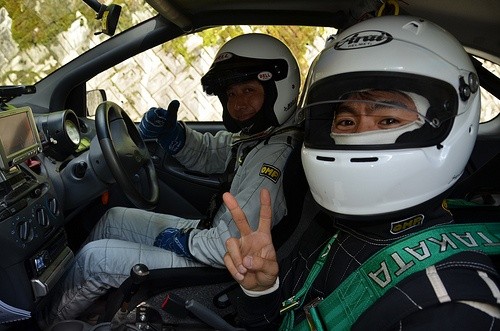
[31,33,302,324]
[91,13,500,331]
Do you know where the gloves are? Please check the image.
[136,100,183,154]
[152,226,193,263]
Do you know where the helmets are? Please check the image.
[298,14,483,218]
[194,32,302,141]
